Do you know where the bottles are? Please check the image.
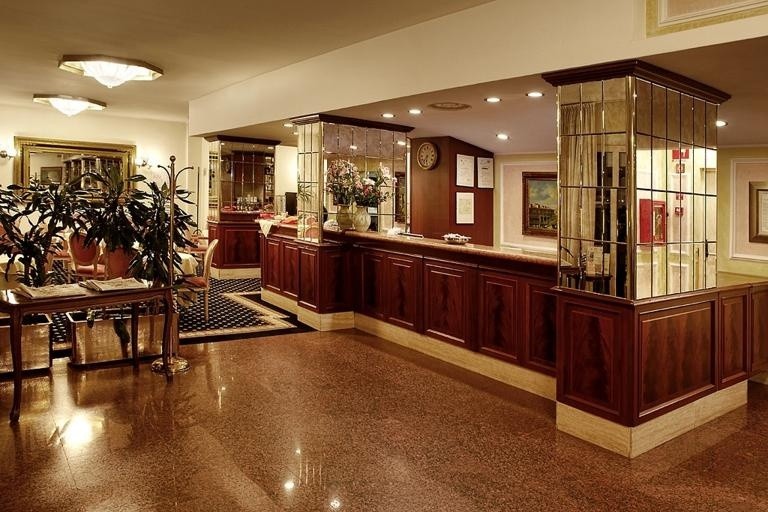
[236,194,259,210]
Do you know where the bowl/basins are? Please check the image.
[272,212,289,222]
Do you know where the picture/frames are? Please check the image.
[729,158,768,263]
[499,161,558,255]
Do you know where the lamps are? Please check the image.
[31,93,107,120]
[54,53,162,89]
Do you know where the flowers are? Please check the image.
[350,165,392,204]
[325,159,361,203]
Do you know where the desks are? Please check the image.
[577,270,613,295]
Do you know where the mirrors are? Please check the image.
[11,136,137,206]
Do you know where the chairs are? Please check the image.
[1,217,219,323]
[561,244,586,289]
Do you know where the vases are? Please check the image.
[348,204,372,231]
[333,206,351,229]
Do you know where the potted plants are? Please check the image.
[1,170,58,372]
[59,156,202,366]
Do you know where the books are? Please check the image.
[10,276,147,301]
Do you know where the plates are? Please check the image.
[443,236,471,244]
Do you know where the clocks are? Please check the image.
[415,141,439,170]
